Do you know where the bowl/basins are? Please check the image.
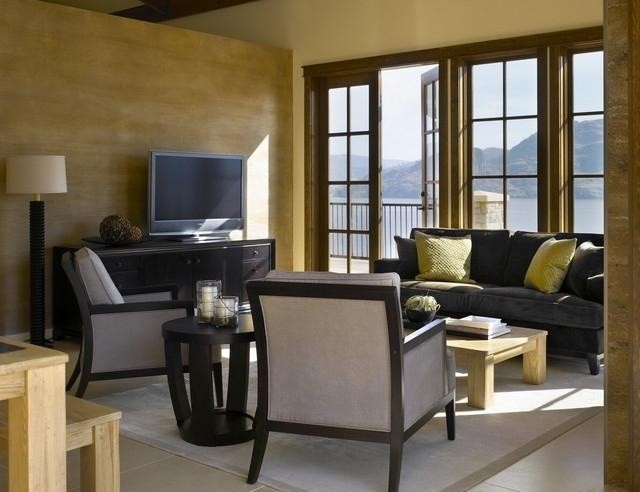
[405,304,441,324]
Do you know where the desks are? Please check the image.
[0,336,69,492]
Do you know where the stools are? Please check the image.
[67,392,123,492]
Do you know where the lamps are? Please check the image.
[6,155,69,349]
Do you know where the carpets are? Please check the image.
[86,354,604,492]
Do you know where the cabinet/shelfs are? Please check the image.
[52,238,276,343]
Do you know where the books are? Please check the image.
[443,315,511,340]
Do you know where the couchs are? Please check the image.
[373,227,604,376]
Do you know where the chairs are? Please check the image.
[246,280,455,492]
[59,250,223,409]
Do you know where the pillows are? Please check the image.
[393,229,604,305]
[72,246,125,306]
[263,271,403,337]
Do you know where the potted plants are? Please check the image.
[406,295,437,322]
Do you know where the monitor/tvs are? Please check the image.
[147,150,246,242]
